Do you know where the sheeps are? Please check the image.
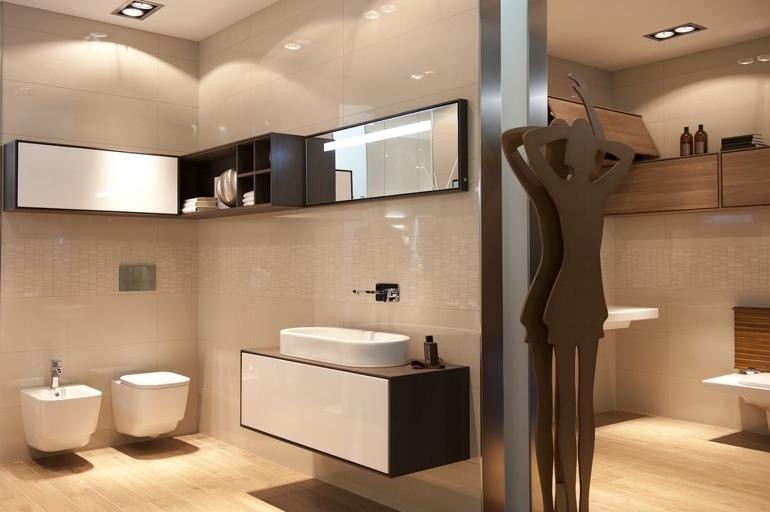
[50,359,65,389]
[385,287,398,302]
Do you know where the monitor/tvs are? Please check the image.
[720,133,766,151]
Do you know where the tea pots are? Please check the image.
[305,97,470,204]
[2,138,182,218]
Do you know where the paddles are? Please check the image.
[249,475,410,512]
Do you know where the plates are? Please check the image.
[593,153,720,218]
[549,96,660,166]
[240,351,474,478]
[175,130,306,217]
[719,146,770,210]
